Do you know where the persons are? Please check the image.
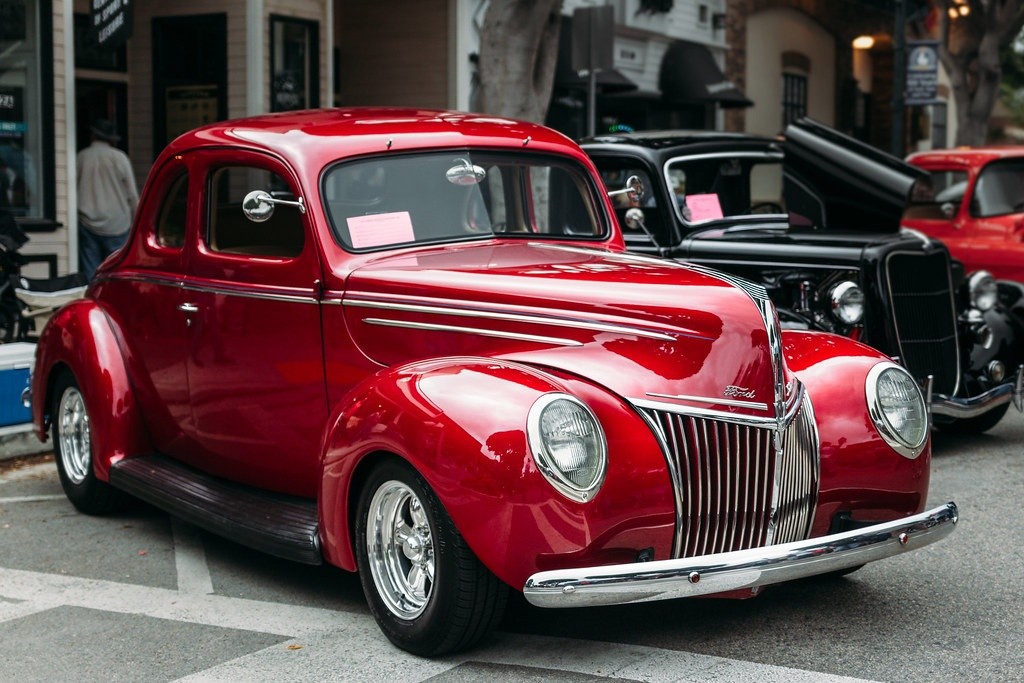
[77,119,140,283]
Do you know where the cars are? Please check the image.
[460,116,1023,434]
[898,143,1024,285]
[19,107,957,658]
